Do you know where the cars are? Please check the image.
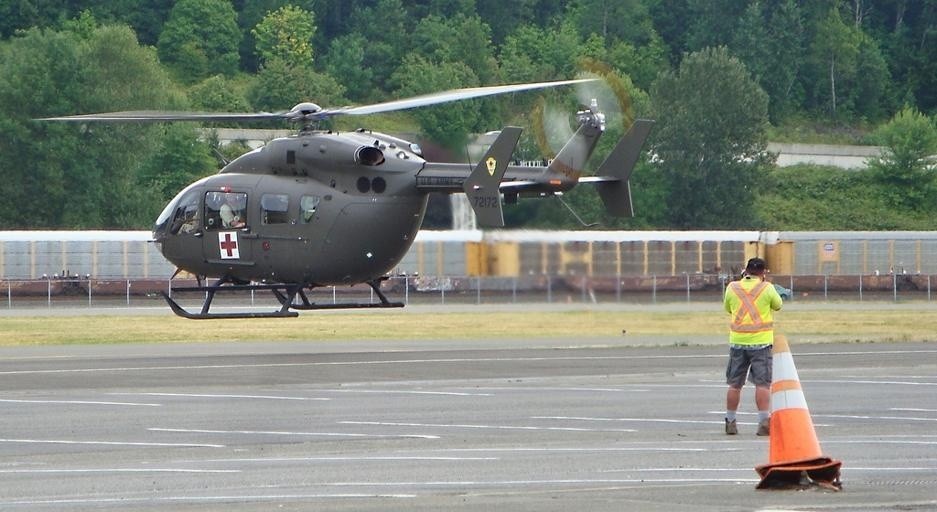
[772,284,791,301]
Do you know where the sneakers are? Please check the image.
[756,418,771,436]
[725,417,738,436]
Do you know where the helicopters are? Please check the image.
[28,56,656,320]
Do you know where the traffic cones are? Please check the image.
[757,334,841,487]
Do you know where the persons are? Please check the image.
[219,193,246,229]
[724,257,783,435]
[178,208,200,234]
